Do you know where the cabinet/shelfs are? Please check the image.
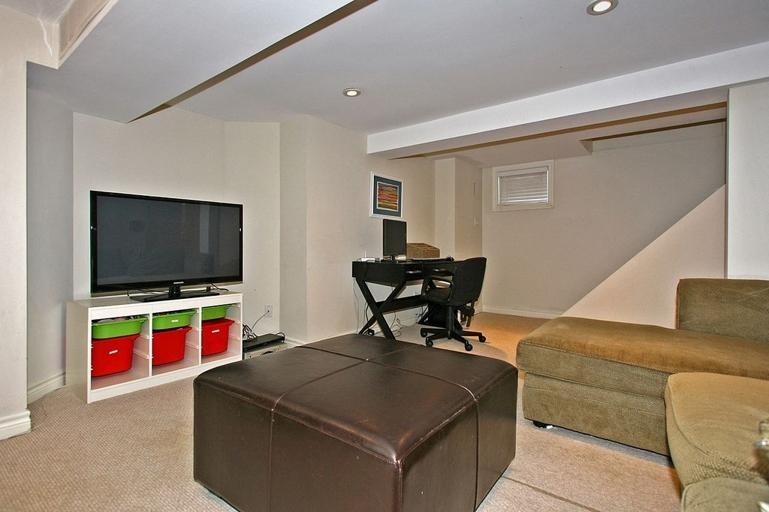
[66,294,243,405]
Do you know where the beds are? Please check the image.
[515,278,769,459]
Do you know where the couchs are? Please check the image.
[664,371,769,512]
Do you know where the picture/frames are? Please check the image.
[369,170,404,223]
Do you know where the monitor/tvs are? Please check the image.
[89,190,244,302]
[381,219,407,263]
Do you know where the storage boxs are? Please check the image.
[202,318,235,356]
[152,326,192,366]
[92,334,141,378]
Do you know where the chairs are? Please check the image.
[420,257,487,351]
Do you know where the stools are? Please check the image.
[193,333,518,512]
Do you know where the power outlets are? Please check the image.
[265,305,273,317]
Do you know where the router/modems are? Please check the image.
[361,251,375,262]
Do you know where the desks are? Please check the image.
[352,259,454,340]
